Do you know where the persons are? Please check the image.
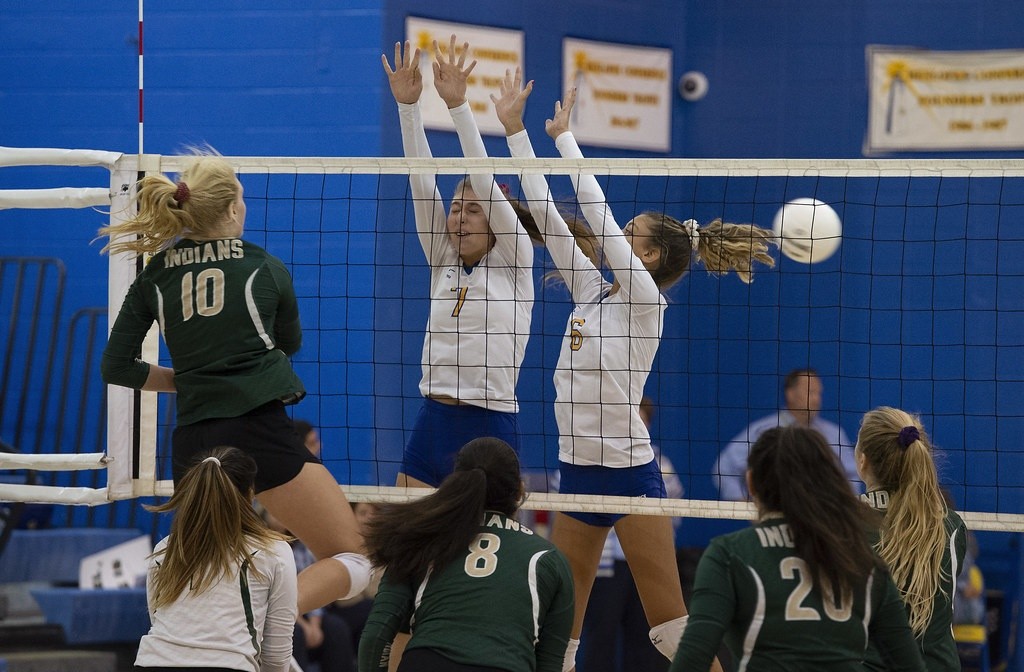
[712,368,860,504]
[489,66,811,672]
[671,426,929,672]
[855,406,969,672]
[943,491,989,672]
[254,421,386,672]
[100,139,374,620]
[545,399,686,672]
[133,446,299,672]
[381,35,606,672]
[357,437,576,672]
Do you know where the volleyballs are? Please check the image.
[773,197,842,265]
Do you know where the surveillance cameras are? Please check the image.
[678,71,709,101]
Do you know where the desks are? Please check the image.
[31,588,150,647]
[0,528,142,585]
